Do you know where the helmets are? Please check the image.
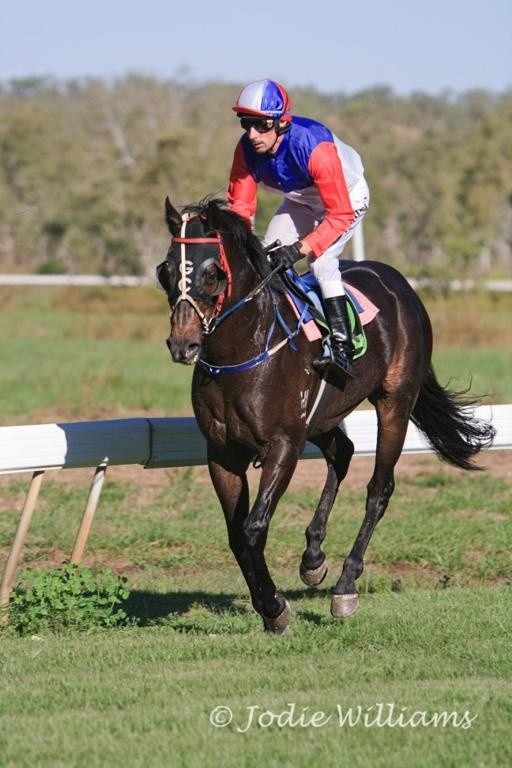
[232,78,293,122]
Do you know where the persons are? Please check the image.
[227,79,372,380]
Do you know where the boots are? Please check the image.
[316,295,356,376]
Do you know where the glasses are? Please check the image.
[236,118,275,135]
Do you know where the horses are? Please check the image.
[156,192,497,637]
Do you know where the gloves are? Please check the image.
[270,241,306,273]
